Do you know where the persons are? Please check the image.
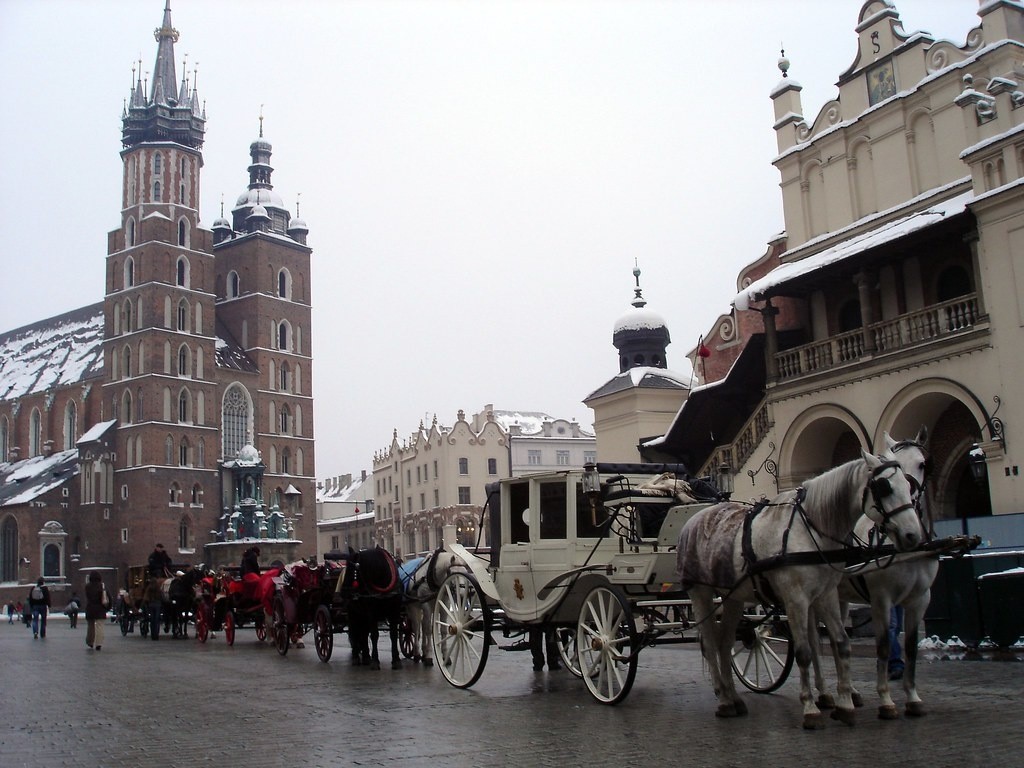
[149,543,173,575]
[84,571,111,650]
[527,626,562,672]
[16,600,23,622]
[883,603,904,680]
[28,576,52,640]
[239,546,262,579]
[23,596,32,628]
[8,600,16,624]
[65,590,80,628]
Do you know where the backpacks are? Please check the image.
[32,585,44,600]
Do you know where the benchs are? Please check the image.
[596,461,732,508]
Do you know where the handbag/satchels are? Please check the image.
[101,583,109,608]
[64,601,79,615]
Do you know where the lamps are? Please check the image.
[970,394,1007,478]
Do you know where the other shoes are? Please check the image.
[71,624,73,628]
[88,643,93,648]
[95,646,102,651]
[74,626,76,629]
[34,632,38,640]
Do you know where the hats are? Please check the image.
[156,543,164,548]
[251,546,260,556]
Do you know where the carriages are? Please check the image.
[430,422,982,732]
[116,544,477,672]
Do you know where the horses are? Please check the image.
[144,562,216,639]
[341,546,468,671]
[675,425,940,726]
